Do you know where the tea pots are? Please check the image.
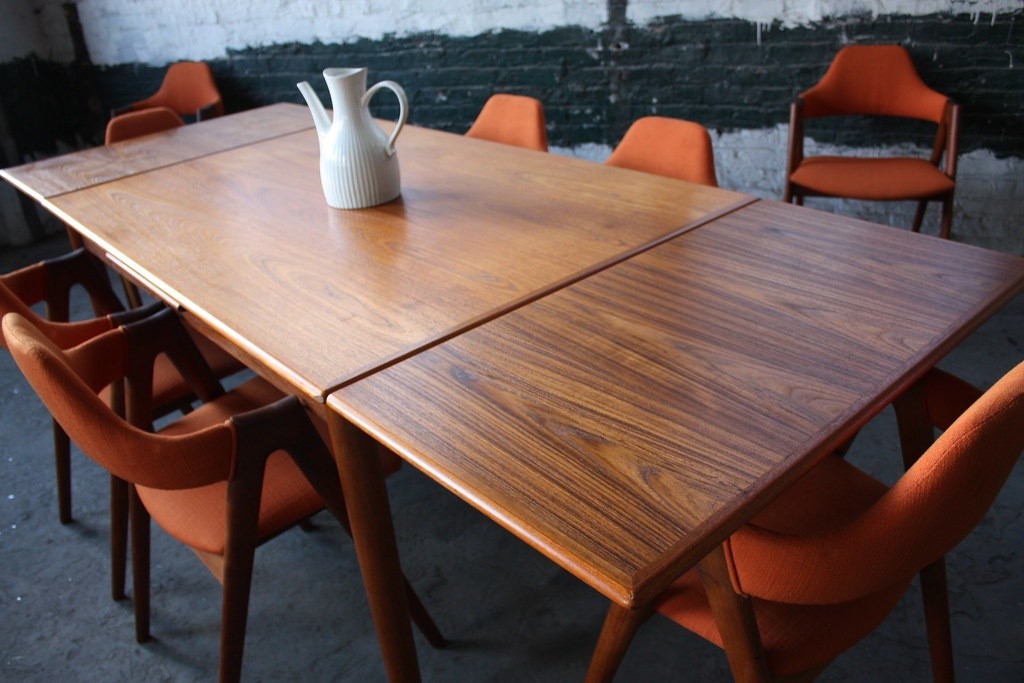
[297,68,408,209]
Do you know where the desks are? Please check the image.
[0,103,334,202]
[42,119,761,473]
[325,199,1024,683]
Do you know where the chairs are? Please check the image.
[0,46,1024,683]
